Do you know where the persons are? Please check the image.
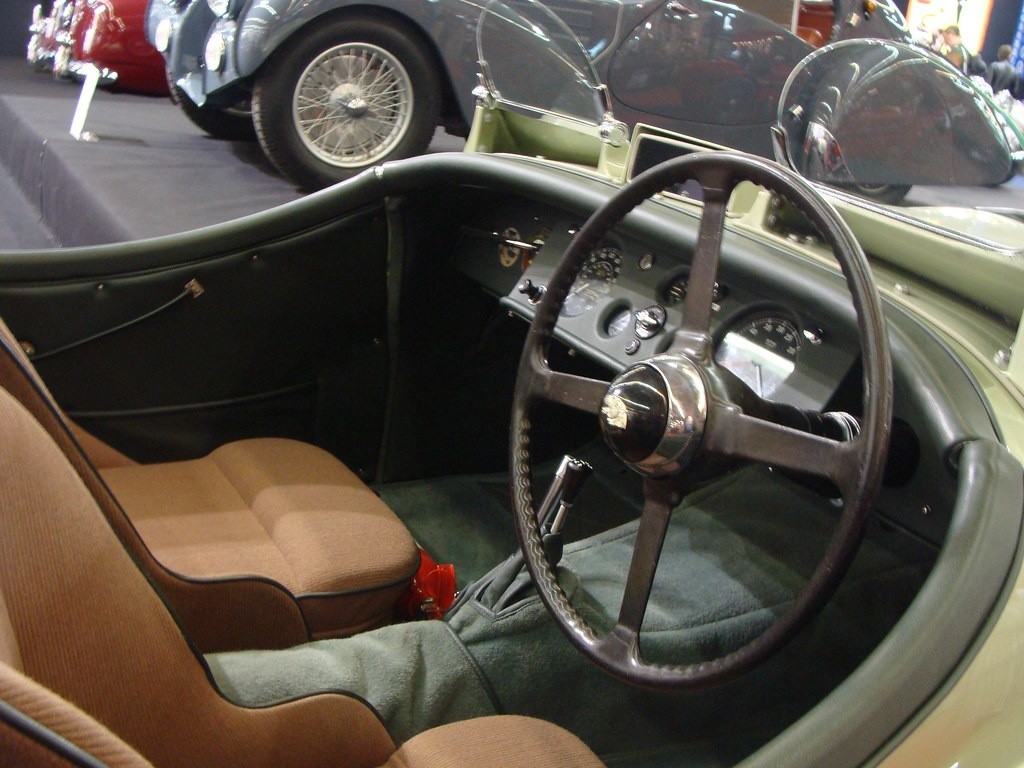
[944,26,968,75]
[984,45,1018,96]
[934,30,949,55]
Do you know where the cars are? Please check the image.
[27,0,173,99]
[142,0,1024,205]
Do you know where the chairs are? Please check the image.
[0,316,422,656]
[0,388,613,768]
